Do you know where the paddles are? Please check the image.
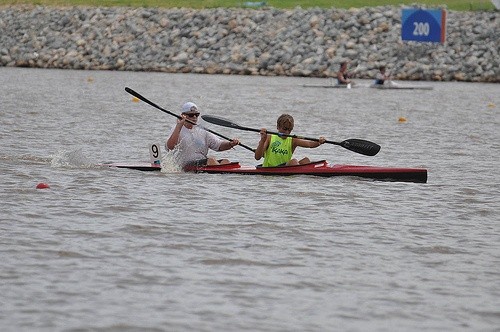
[201,113,382,157]
[123,86,264,159]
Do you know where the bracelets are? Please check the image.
[228,141,236,150]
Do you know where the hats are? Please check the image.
[181,102,199,112]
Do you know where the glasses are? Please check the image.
[186,113,200,117]
[283,127,293,131]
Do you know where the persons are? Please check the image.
[255,114,325,168]
[165,102,240,167]
[338,62,352,86]
[376,66,389,85]
